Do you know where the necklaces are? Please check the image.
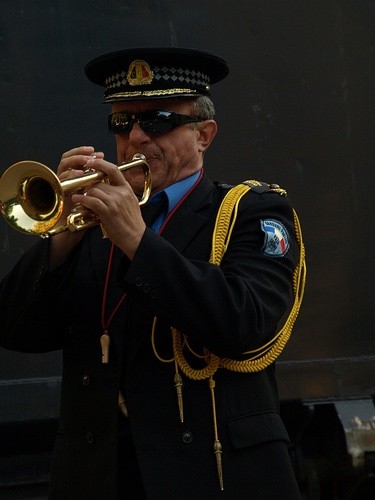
[99,165,204,363]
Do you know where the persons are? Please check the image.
[0,49,305,499]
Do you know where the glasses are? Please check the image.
[107,109,204,135]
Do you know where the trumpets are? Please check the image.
[1,153,153,239]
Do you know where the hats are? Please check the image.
[85,46,230,105]
